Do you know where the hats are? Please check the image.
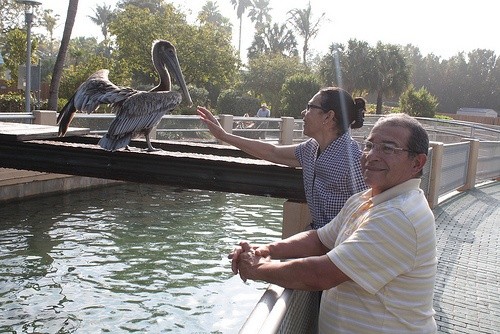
[261,103,269,109]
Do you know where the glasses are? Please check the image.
[305,103,327,112]
[356,139,419,155]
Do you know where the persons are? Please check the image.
[255,102,271,141]
[196,86,376,232]
[228,113,438,334]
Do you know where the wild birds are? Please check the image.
[56,39,193,153]
